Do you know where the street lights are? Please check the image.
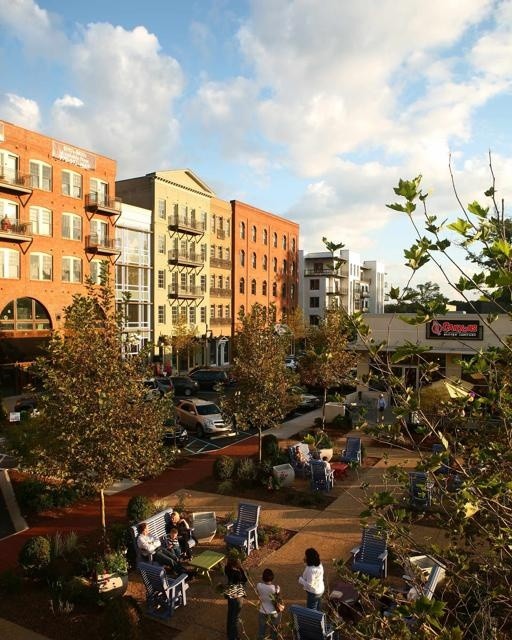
[204,323,216,366]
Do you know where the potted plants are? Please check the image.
[71,532,133,613]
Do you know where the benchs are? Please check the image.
[289,440,322,481]
[129,508,196,568]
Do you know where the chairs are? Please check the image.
[191,511,217,551]
[350,524,389,580]
[309,459,335,495]
[409,471,437,510]
[137,561,190,620]
[287,604,341,639]
[341,436,362,468]
[222,501,262,556]
[382,562,443,632]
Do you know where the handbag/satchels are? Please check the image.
[276,599,285,612]
[188,539,196,548]
[224,584,246,599]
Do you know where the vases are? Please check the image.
[246,479,258,489]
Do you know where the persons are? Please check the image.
[164,529,182,560]
[256,568,280,640]
[298,547,326,610]
[384,582,423,606]
[136,523,178,573]
[169,512,194,560]
[223,555,250,640]
[317,457,321,465]
[321,456,331,475]
[295,446,311,468]
[376,394,387,423]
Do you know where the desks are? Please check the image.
[189,549,226,586]
[331,463,349,481]
